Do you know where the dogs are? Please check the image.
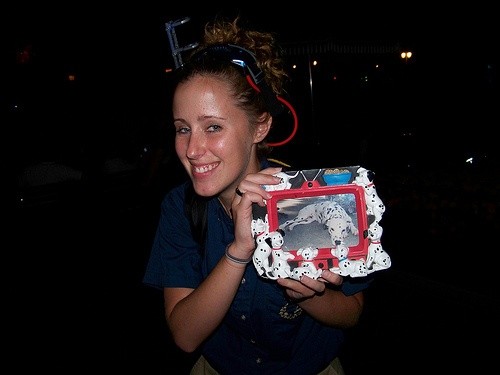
[284,246,322,281]
[350,167,386,224]
[362,223,392,273]
[264,228,295,281]
[328,244,367,278]
[276,199,359,245]
[250,216,274,277]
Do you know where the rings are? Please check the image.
[235,187,243,196]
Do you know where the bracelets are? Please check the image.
[225,243,251,263]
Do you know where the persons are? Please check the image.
[146,21,370,375]
